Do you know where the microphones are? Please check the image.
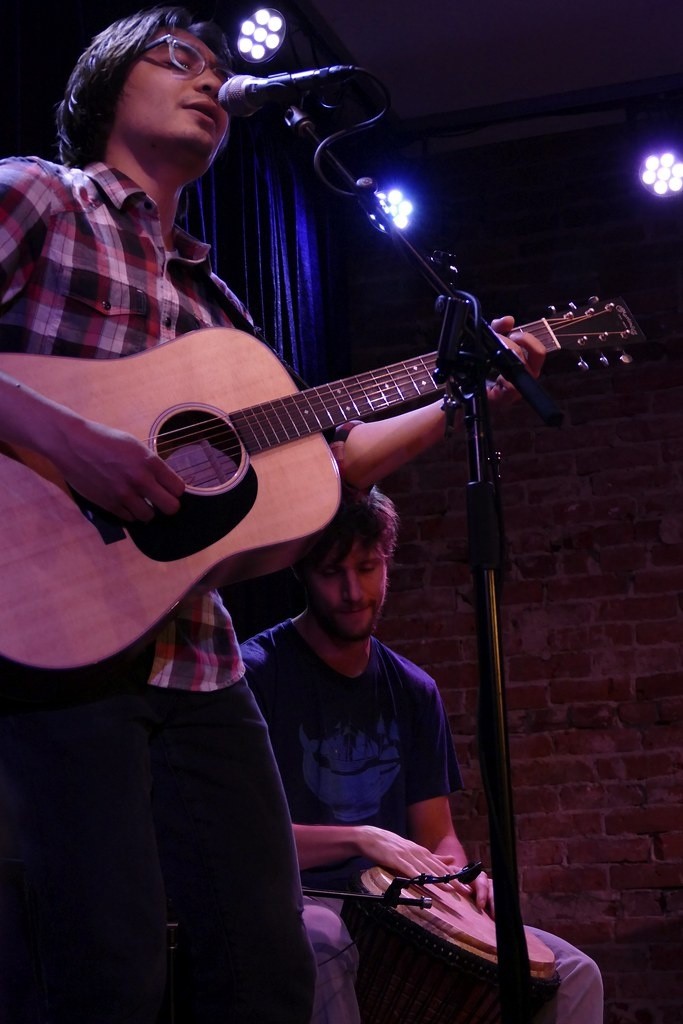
[459,861,483,884]
[218,65,354,118]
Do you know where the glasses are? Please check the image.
[134,34,241,85]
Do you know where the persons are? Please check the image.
[235,484,606,1024]
[2,0,547,1024]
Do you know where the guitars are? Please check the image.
[0,295,645,699]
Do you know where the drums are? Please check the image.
[338,861,562,1024]
